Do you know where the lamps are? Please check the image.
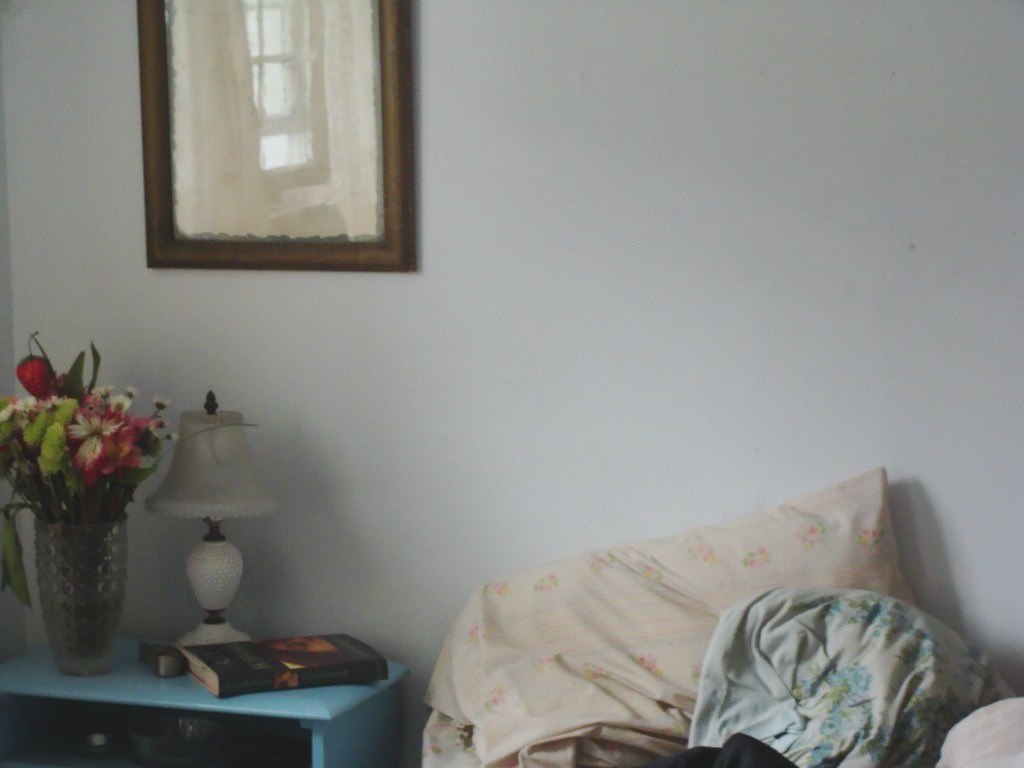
[143,391,253,649]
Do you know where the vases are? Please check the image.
[32,520,126,677]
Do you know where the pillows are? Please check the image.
[423,464,916,768]
[686,585,1017,768]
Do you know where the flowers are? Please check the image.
[0,332,259,616]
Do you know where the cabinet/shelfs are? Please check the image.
[0,634,412,768]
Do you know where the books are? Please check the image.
[175,632,389,700]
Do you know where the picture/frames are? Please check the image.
[133,0,421,274]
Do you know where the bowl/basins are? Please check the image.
[55,716,129,759]
[129,716,229,768]
